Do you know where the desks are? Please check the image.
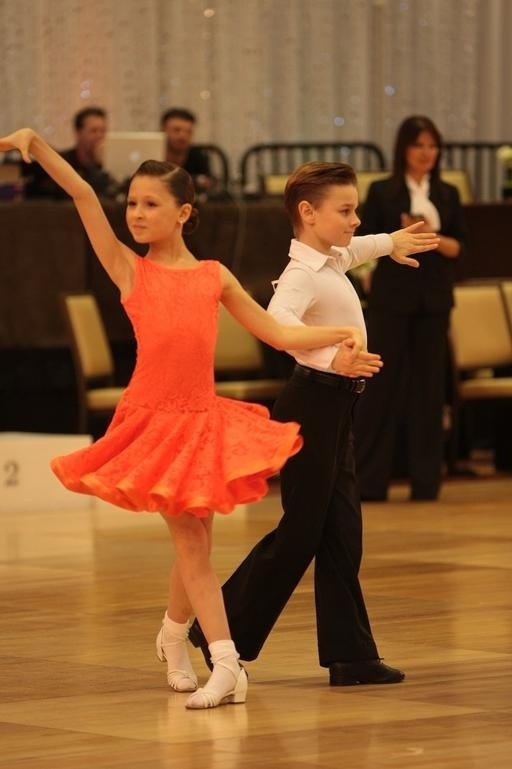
[0,196,511,348]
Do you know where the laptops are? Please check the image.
[102,132,167,183]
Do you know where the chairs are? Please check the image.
[258,168,475,209]
[63,278,512,445]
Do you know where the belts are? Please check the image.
[294,362,369,395]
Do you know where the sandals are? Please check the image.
[184,654,250,709]
[154,618,198,692]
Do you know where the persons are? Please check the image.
[1,127,362,710]
[186,162,443,684]
[351,113,465,502]
[160,107,212,202]
[26,105,114,198]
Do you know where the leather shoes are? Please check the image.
[187,615,249,682]
[328,657,406,686]
